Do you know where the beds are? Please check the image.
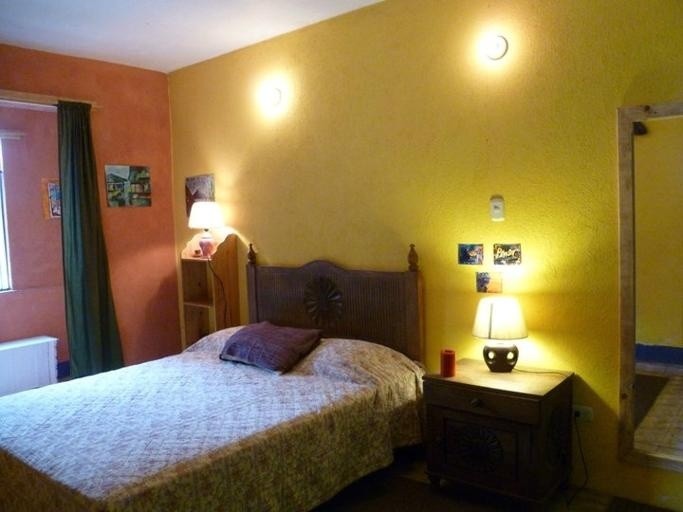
[0,242,431,512]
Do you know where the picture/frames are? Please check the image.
[41,176,64,221]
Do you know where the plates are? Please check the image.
[192,254,202,257]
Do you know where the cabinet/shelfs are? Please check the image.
[176,234,241,351]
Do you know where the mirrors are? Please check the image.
[616,100,683,476]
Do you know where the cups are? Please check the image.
[439,349,457,377]
[194,250,200,254]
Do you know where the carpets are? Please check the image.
[634,373,670,430]
[312,472,529,512]
[605,496,678,512]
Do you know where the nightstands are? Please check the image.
[421,358,574,512]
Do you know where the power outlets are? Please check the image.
[572,405,593,424]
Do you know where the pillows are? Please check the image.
[218,321,324,375]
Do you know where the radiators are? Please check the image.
[0,335,60,398]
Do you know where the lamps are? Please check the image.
[471,296,528,372]
[187,201,223,259]
